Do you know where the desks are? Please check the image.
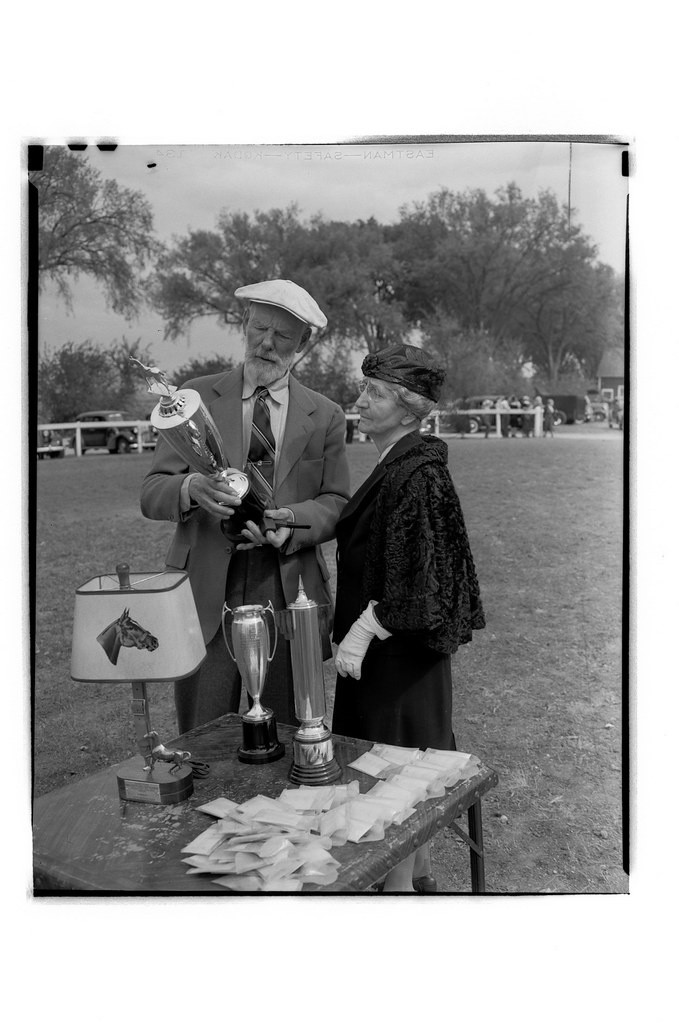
[33,713,499,891]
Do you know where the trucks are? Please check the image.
[531,387,587,426]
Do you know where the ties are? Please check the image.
[247,387,277,498]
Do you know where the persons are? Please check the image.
[533,396,543,409]
[331,344,486,891]
[481,399,494,438]
[496,397,511,436]
[543,398,556,437]
[139,279,350,733]
[509,396,522,437]
[521,396,535,437]
[455,395,471,438]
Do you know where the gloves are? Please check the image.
[334,598,393,681]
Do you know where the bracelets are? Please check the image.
[373,612,383,628]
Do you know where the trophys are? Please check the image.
[221,599,286,765]
[288,575,342,785]
[130,355,268,546]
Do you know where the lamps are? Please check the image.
[68,561,207,805]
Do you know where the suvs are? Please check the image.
[423,395,505,434]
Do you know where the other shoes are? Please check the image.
[376,874,437,891]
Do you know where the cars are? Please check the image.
[587,388,609,422]
[608,396,624,430]
[66,410,159,456]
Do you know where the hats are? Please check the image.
[360,344,448,403]
[234,277,328,334]
